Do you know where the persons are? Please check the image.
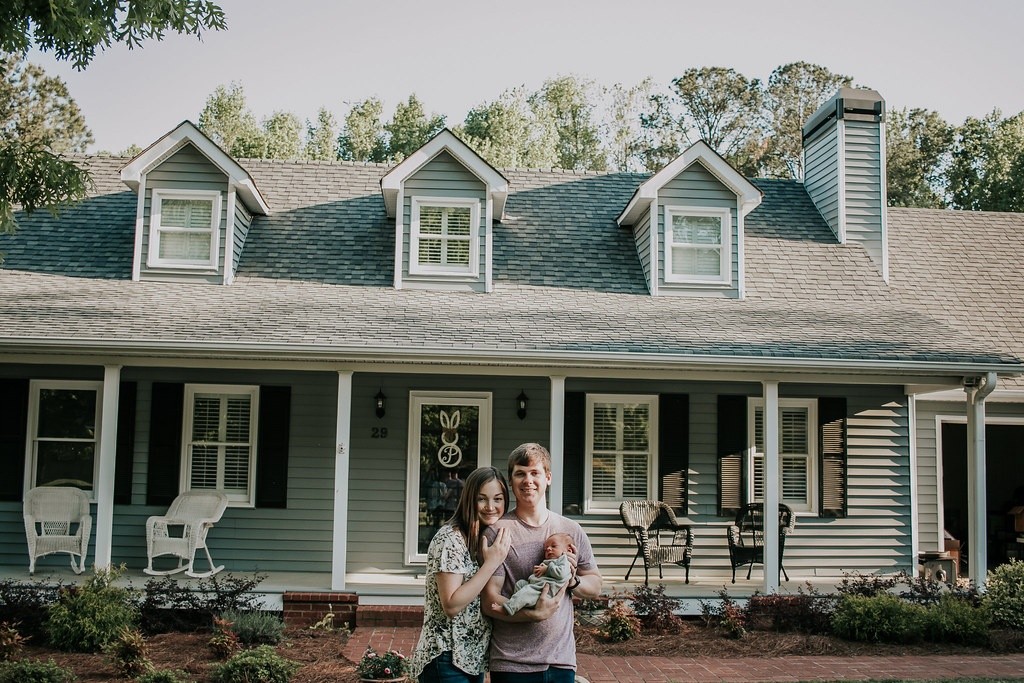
[426,469,466,532]
[490,533,575,616]
[477,442,602,683]
[411,467,511,683]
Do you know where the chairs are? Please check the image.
[143,489,228,577]
[24,486,94,572]
[728,500,796,587]
[620,498,693,587]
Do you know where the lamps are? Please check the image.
[517,392,529,420]
[374,388,387,418]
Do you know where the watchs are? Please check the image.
[567,575,580,589]
